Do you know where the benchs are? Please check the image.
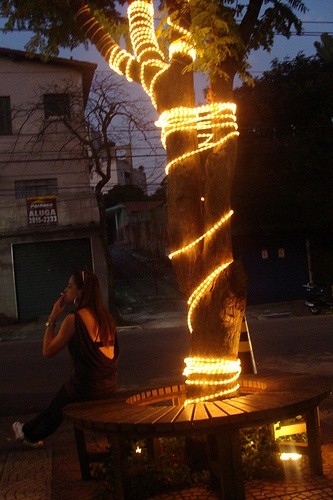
[62,370,330,500]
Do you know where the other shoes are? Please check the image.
[19,440,44,449]
[12,422,24,441]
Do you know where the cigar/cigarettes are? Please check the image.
[60,292,63,295]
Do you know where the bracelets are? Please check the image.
[49,314,56,321]
[45,322,55,327]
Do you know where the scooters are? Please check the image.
[302,283,333,316]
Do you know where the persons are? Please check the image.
[12,271,121,449]
